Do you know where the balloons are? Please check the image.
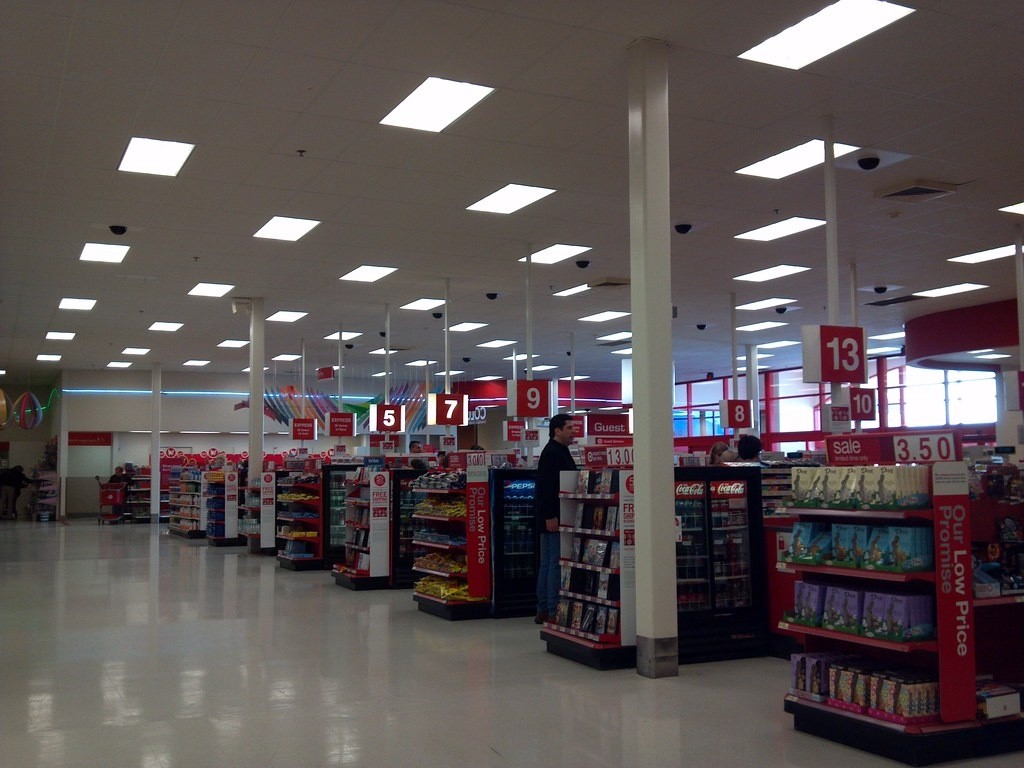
[0,386,62,430]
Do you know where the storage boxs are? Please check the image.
[791,651,941,717]
[976,668,1020,719]
[974,568,1000,598]
[789,523,935,574]
[792,465,929,510]
[795,580,934,635]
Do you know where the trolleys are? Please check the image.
[98,482,132,525]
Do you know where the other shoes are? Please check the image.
[535,612,548,623]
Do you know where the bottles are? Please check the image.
[504,489,535,542]
[673,496,752,611]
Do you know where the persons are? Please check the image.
[409,440,421,453]
[108,466,134,524]
[471,445,484,450]
[707,436,761,465]
[535,415,577,622]
[0,466,33,520]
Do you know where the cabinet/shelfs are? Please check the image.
[127,467,821,670]
[28,477,57,521]
[775,461,1024,768]
